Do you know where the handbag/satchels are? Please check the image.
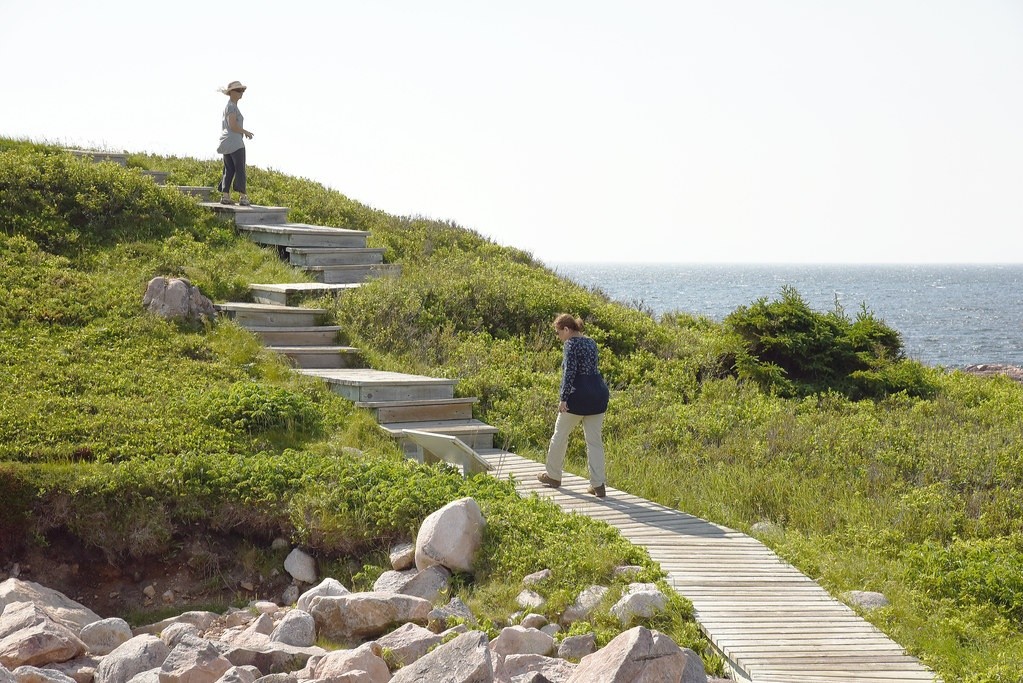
[564,374,609,415]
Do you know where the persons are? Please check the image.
[537,314,609,497]
[217,81,254,206]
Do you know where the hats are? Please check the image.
[222,81,247,95]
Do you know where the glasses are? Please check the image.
[232,89,244,93]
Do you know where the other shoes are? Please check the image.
[537,472,561,488]
[239,195,250,205]
[587,483,605,497]
[220,194,235,204]
